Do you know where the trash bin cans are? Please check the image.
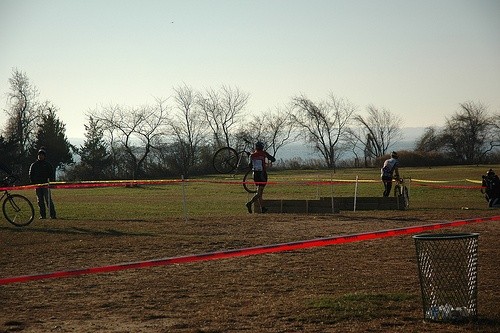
[412,232,480,324]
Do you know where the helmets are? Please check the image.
[255,142,265,149]
[391,151,399,157]
[486,171,493,176]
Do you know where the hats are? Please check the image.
[38,146,46,155]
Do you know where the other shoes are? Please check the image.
[39,216,46,219]
[50,216,57,219]
[489,198,494,207]
[245,203,252,213]
[261,206,269,213]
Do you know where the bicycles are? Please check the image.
[0,175,35,226]
[212,139,258,193]
[394,177,410,210]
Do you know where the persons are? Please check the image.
[28,146,57,219]
[245,141,275,213]
[380,151,401,197]
